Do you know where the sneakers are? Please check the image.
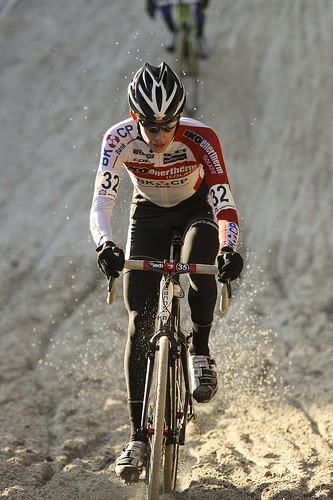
[189,347,219,404]
[115,439,148,481]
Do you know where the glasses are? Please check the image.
[138,116,178,133]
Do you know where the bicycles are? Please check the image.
[105,228,232,500]
[153,0,207,119]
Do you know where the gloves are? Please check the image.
[216,245,243,285]
[95,241,125,280]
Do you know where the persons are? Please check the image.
[89,62,244,479]
[145,0,212,59]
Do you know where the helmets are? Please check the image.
[127,61,187,121]
